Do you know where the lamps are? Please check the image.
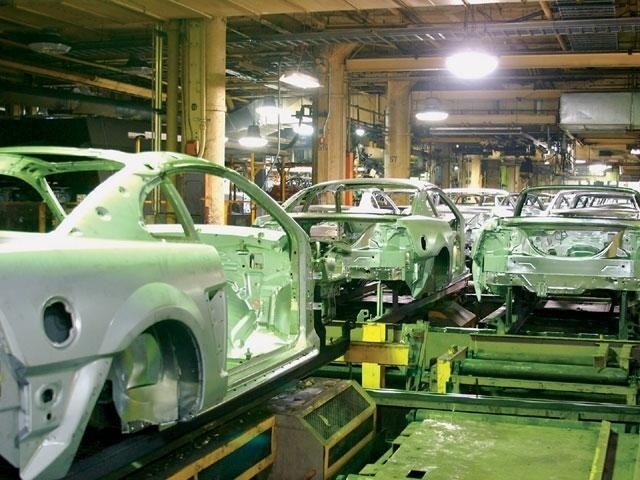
[279,42,325,93]
[415,94,451,124]
[254,96,293,121]
[237,126,269,147]
[445,20,502,82]
